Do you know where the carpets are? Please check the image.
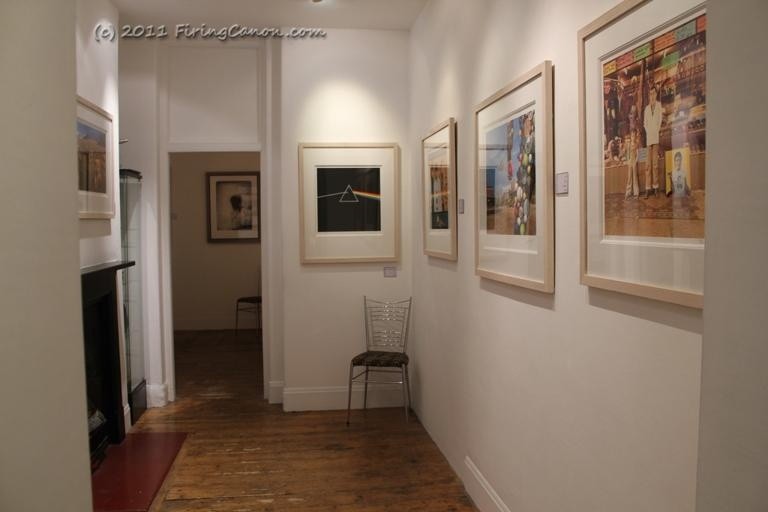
[92,431,188,512]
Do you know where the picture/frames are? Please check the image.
[76,94,117,220]
[299,142,400,266]
[418,0,703,311]
[205,172,260,244]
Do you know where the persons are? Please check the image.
[623,127,639,202]
[666,150,691,201]
[642,86,664,200]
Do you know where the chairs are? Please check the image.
[235,296,261,337]
[346,295,412,428]
